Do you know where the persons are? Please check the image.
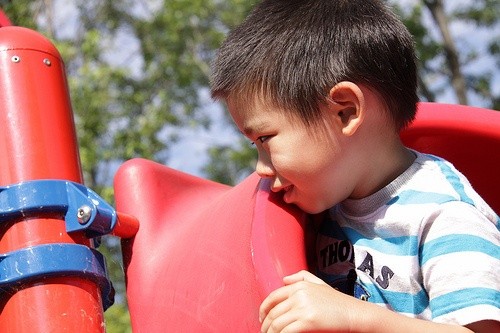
[210,1,500,333]
